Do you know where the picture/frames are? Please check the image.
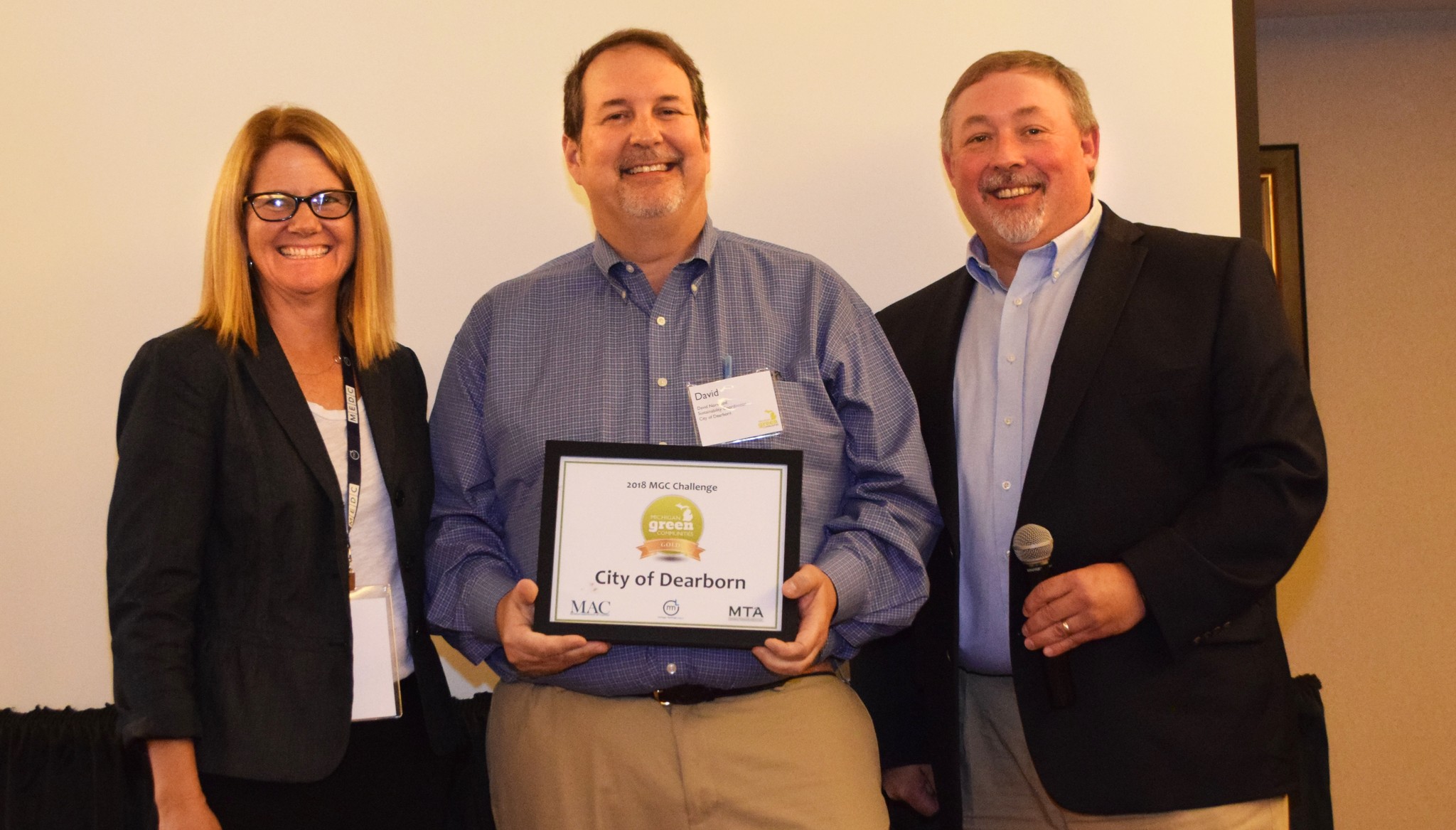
[1263,145,1309,377]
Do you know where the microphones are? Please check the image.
[1011,523,1076,707]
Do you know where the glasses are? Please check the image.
[243,189,357,222]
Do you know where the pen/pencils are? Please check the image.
[724,355,732,379]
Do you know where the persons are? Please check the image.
[426,30,942,829]
[105,109,455,830]
[848,50,1329,829]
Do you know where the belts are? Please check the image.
[636,658,836,705]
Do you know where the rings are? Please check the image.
[1061,619,1072,636]
